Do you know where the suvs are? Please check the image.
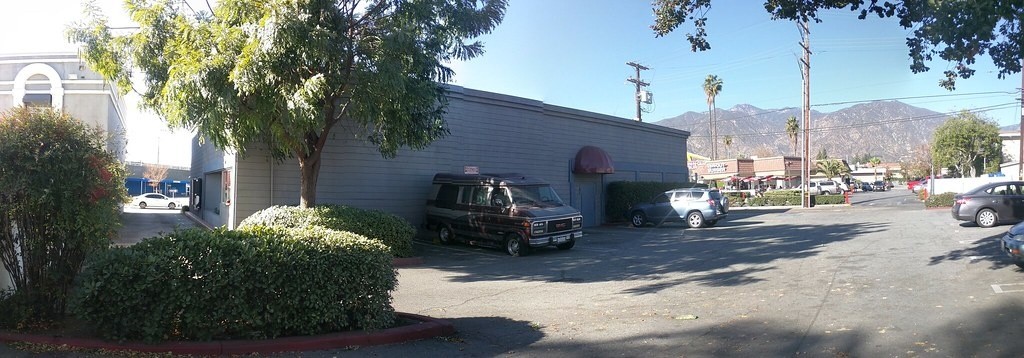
[628,188,729,228]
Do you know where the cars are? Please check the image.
[869,183,874,188]
[853,182,872,191]
[872,181,886,192]
[951,180,1024,228]
[1001,221,1024,269]
[850,181,859,189]
[837,182,850,194]
[132,193,182,209]
[912,181,928,194]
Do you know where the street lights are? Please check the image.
[707,97,713,160]
[785,161,793,188]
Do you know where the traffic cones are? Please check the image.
[845,192,850,204]
[923,188,927,200]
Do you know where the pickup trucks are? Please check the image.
[907,176,942,190]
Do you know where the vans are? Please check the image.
[797,180,839,196]
[425,172,583,257]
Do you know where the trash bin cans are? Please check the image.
[810,194,816,207]
